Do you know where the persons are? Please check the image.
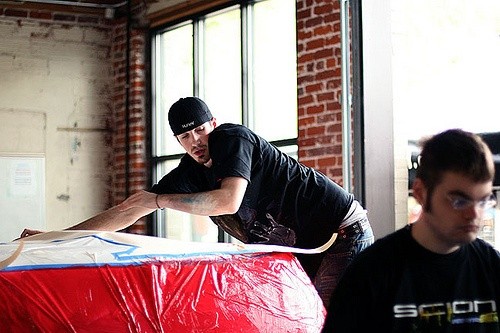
[21,96,376,306]
[320,129,500,333]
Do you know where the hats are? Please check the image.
[167,97,213,137]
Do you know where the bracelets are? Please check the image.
[155,194,164,210]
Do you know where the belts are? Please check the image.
[336,221,362,241]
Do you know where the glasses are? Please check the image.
[440,187,497,210]
